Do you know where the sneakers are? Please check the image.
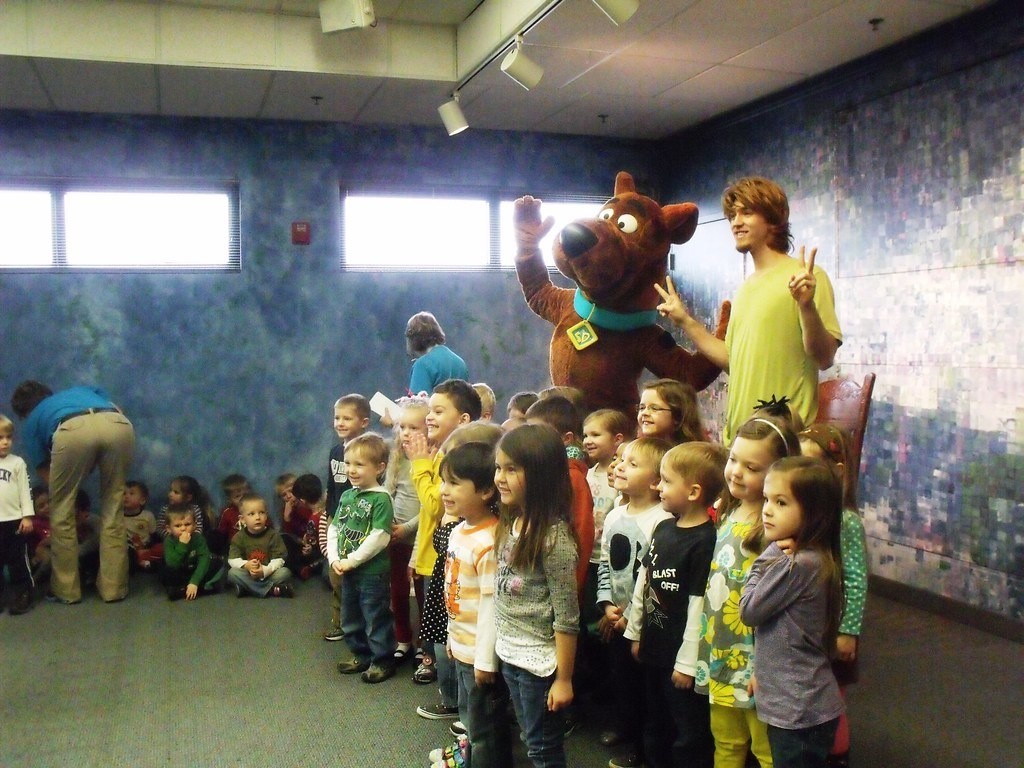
[429,739,469,762]
[430,748,467,768]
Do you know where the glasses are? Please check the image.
[634,403,676,415]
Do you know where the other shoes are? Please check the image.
[236,583,246,598]
[416,703,460,719]
[337,656,370,673]
[49,593,81,604]
[168,586,186,601]
[599,733,619,745]
[825,757,848,768]
[394,646,412,665]
[413,663,436,682]
[414,651,424,668]
[450,722,467,736]
[301,565,312,579]
[608,751,649,768]
[361,662,396,682]
[10,592,33,613]
[563,720,577,738]
[833,661,850,685]
[273,582,287,597]
[324,627,345,640]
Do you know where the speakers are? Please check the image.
[319,0,375,33]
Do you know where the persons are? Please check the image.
[0,414,35,615]
[380,311,469,429]
[27,461,324,600]
[11,380,135,605]
[319,378,870,768]
[654,176,843,446]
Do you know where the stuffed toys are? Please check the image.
[514,172,731,421]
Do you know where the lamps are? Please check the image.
[499,34,545,91]
[437,90,469,137]
[593,0,640,28]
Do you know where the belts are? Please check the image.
[55,408,119,425]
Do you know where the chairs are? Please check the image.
[816,372,878,488]
